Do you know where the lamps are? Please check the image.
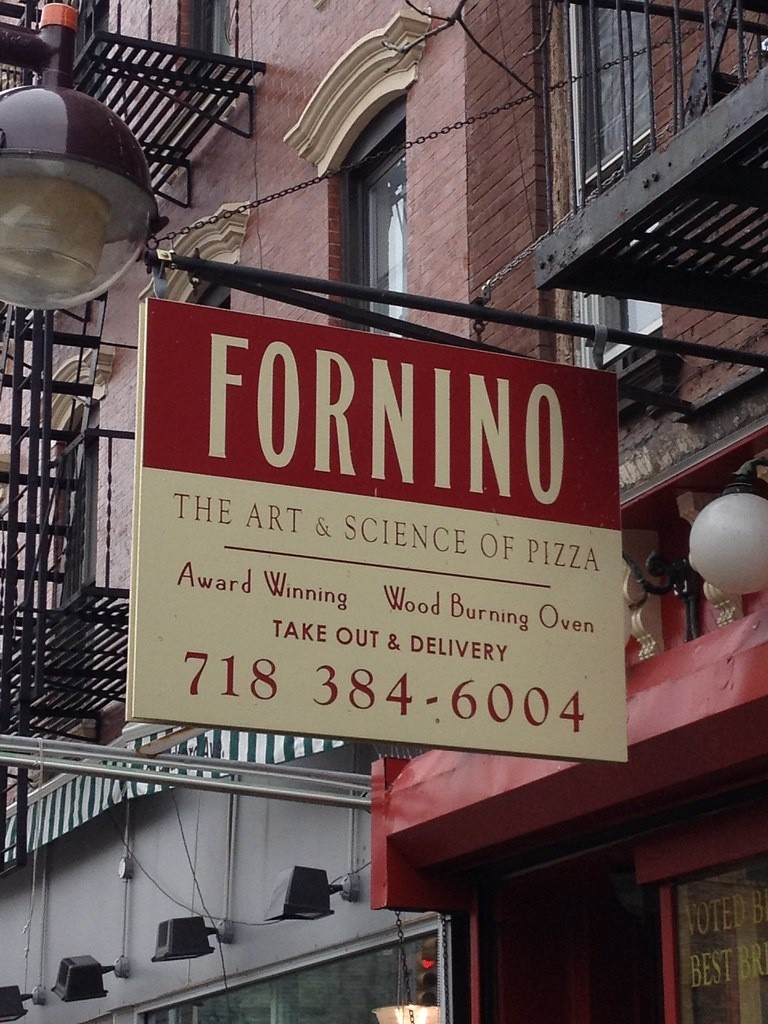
[689,457,768,593]
[265,866,361,922]
[0,984,45,1022]
[371,946,438,1024]
[153,916,235,963]
[51,954,131,1002]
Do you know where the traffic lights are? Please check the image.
[414,946,436,1004]
